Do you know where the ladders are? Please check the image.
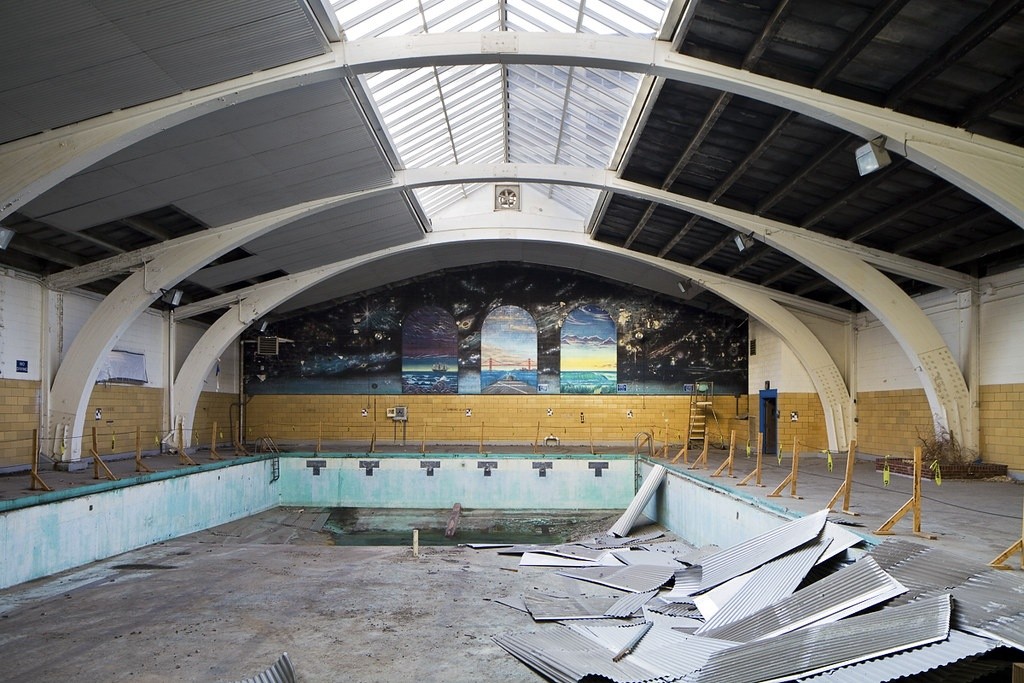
[687,382,725,449]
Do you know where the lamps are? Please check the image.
[734,231,755,253]
[634,331,644,340]
[676,278,692,294]
[855,134,892,177]
[0,223,18,252]
[252,318,269,333]
[159,288,184,307]
